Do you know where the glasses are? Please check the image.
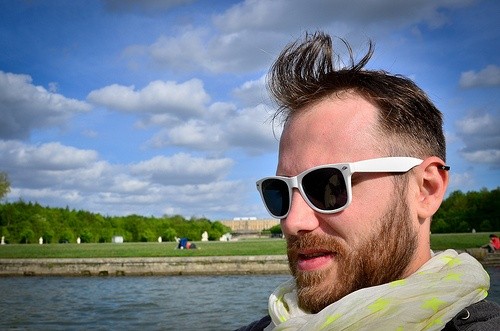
[256,157,423,220]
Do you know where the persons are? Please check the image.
[234,31,500,331]
[489,234,500,253]
